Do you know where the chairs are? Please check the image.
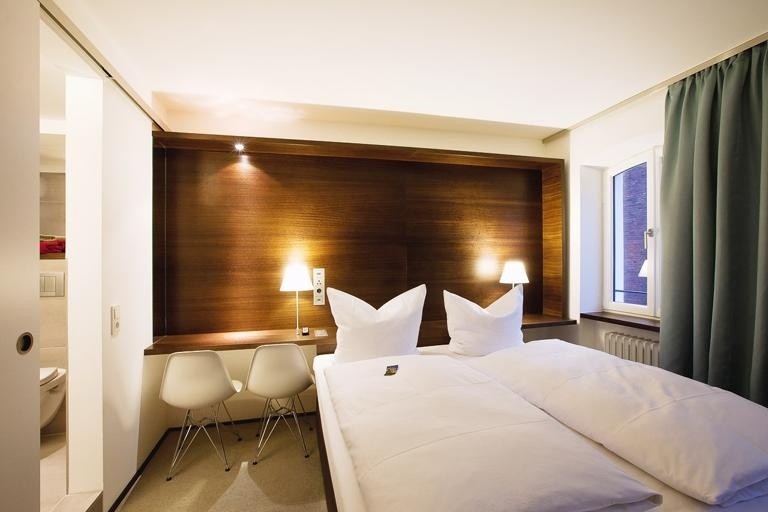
[157,343,316,481]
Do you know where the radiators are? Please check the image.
[603,331,659,367]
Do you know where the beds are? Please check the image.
[312,341,767,512]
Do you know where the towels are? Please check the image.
[40,239,64,255]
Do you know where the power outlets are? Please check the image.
[311,268,325,306]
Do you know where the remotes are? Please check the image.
[302,327,308,334]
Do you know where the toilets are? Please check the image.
[40,366,67,431]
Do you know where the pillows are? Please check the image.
[325,283,525,361]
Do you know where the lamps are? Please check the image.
[280,266,313,336]
[498,261,529,290]
